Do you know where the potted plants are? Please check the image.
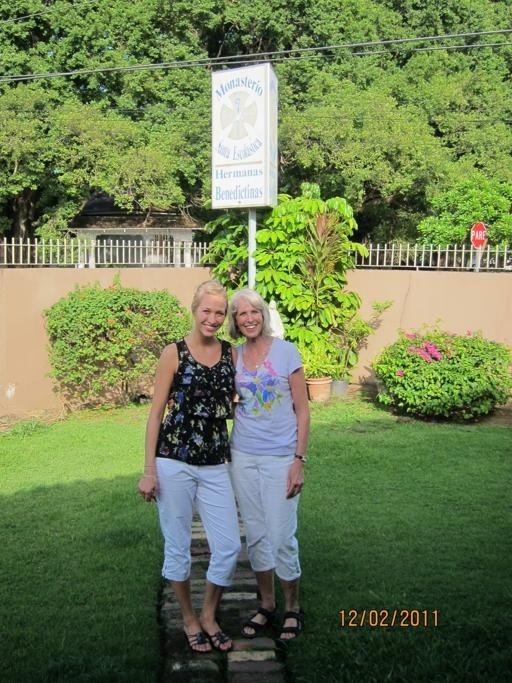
[285,325,356,402]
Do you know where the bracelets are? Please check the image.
[295,453,307,463]
[143,475,156,479]
[144,465,155,468]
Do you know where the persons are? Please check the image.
[226,286,312,643]
[137,278,243,654]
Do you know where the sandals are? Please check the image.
[241,607,306,638]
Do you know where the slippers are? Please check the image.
[184,629,233,654]
